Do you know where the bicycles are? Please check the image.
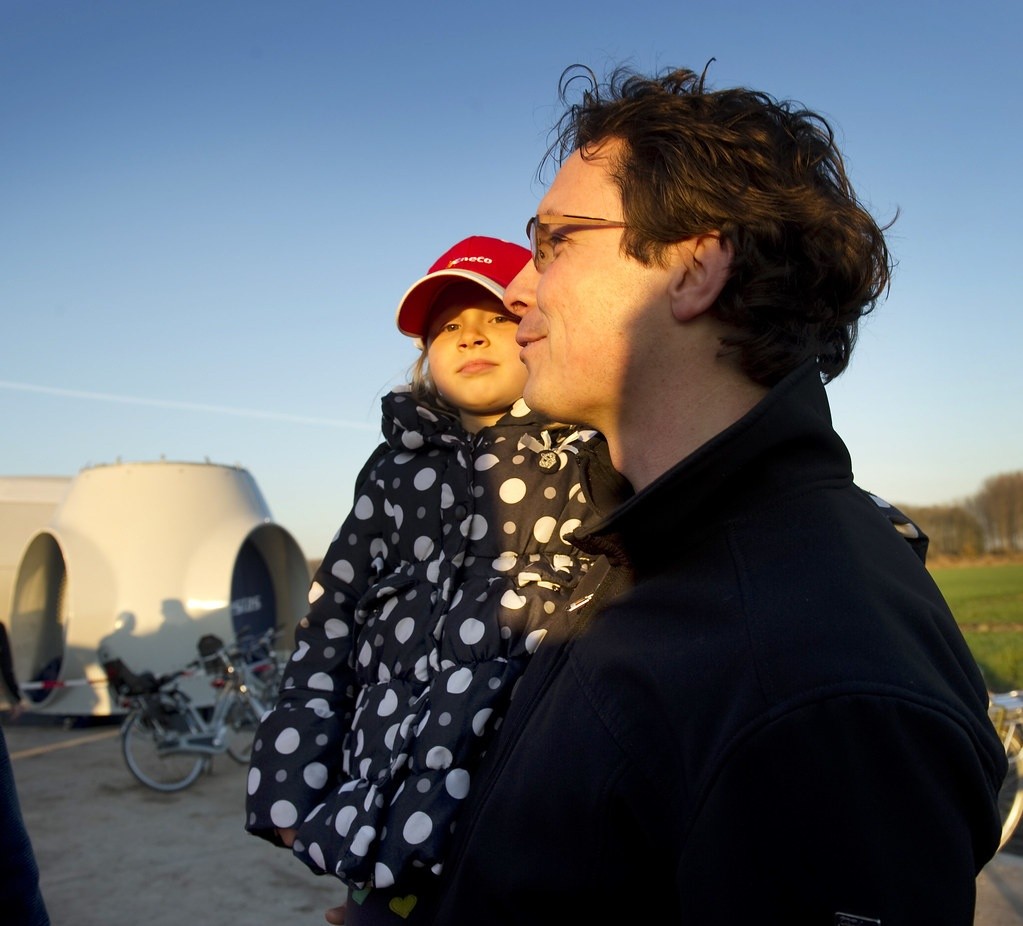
[113,619,295,793]
[984,690,1023,856]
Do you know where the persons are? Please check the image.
[244,56,1008,926]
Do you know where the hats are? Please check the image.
[395,235,534,338]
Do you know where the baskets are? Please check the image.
[223,646,279,690]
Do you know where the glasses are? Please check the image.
[525,213,629,273]
